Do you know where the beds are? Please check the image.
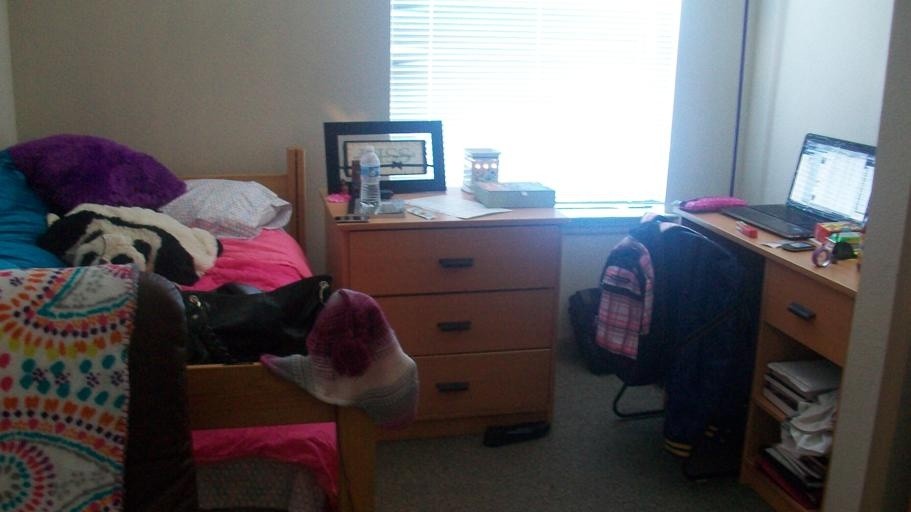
[0,145,377,512]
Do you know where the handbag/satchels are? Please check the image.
[570,285,600,340]
[182,273,333,364]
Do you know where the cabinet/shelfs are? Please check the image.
[318,186,569,441]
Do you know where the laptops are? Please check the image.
[720,132,878,240]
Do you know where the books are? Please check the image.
[761,359,845,511]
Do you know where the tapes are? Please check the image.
[813,247,831,267]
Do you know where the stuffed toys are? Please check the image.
[39,202,224,288]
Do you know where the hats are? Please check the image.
[261,289,418,429]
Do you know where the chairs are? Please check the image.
[0,264,197,512]
[594,223,747,420]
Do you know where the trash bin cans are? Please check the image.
[568,287,633,377]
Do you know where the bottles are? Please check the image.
[360,145,381,218]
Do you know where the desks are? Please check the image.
[672,206,862,512]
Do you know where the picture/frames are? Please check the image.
[324,121,446,195]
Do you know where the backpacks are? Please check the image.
[593,222,664,360]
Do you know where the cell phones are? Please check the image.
[782,242,815,252]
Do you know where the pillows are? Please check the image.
[4,134,187,217]
[158,179,293,239]
